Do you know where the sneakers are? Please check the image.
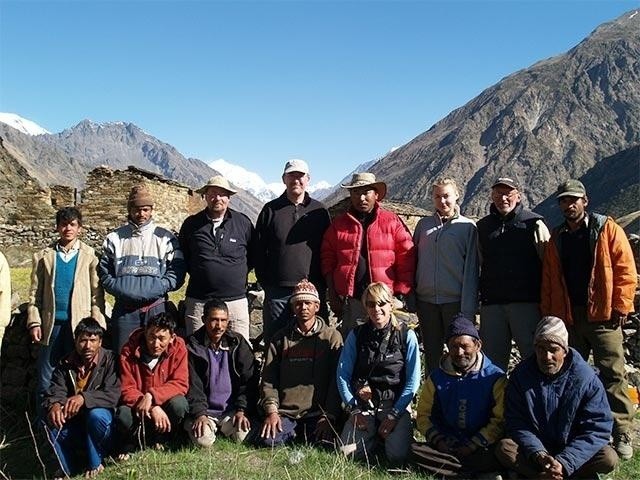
[612,430,634,461]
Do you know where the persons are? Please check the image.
[412,176,479,380]
[320,173,417,325]
[336,281,423,465]
[100,186,187,362]
[255,278,344,447]
[415,313,509,479]
[178,175,258,340]
[475,178,551,374]
[495,316,619,480]
[0,252,11,347]
[182,298,259,449]
[26,206,106,431]
[255,159,333,345]
[540,179,638,461]
[41,316,122,478]
[115,311,189,450]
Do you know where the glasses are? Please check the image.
[365,300,388,309]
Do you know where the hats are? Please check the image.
[443,313,481,341]
[340,172,387,203]
[127,185,154,208]
[289,277,321,304]
[555,178,587,200]
[491,174,520,190]
[283,158,309,175]
[193,175,238,196]
[533,315,570,354]
